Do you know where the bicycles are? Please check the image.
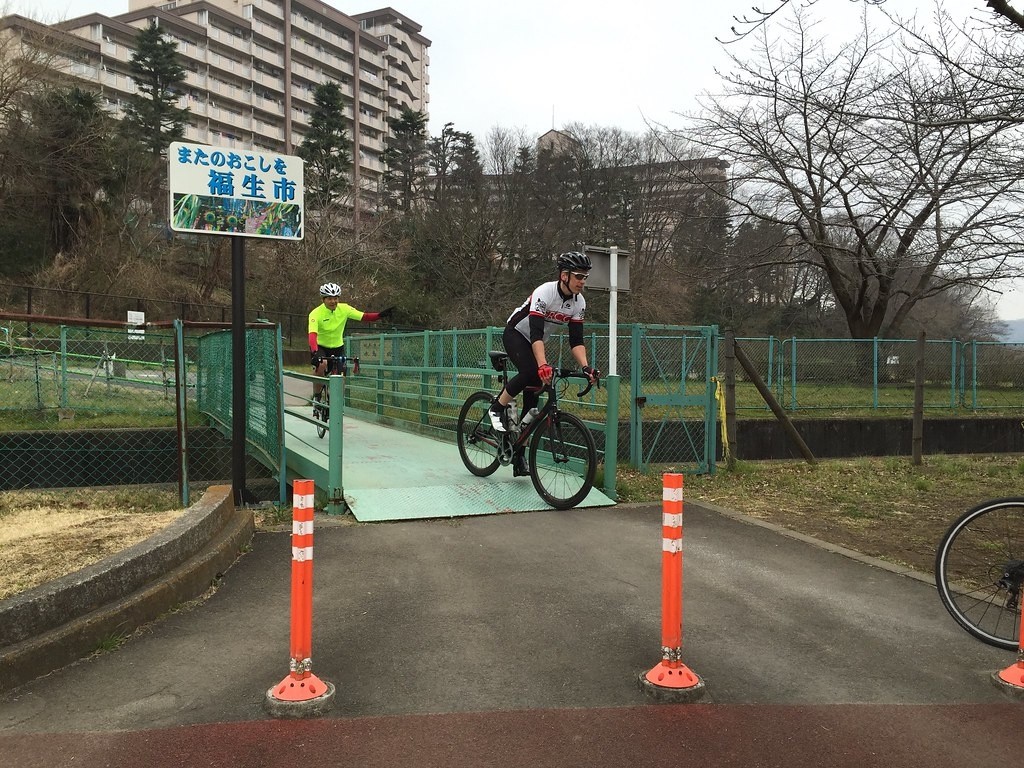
[457,351,600,509]
[936,496,1024,650]
[313,355,360,438]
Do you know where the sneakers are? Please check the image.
[513,466,531,476]
[488,410,507,432]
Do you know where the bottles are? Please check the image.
[518,408,540,432]
[507,397,517,431]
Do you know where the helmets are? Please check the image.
[558,251,592,271]
[319,283,341,298]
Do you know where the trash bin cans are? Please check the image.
[887,355,900,380]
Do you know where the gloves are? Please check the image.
[378,306,397,318]
[311,351,324,368]
[581,366,601,385]
[538,364,553,385]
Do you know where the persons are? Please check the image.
[308,283,395,420]
[488,251,601,477]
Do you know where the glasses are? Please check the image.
[562,271,589,281]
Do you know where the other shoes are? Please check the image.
[313,397,321,406]
